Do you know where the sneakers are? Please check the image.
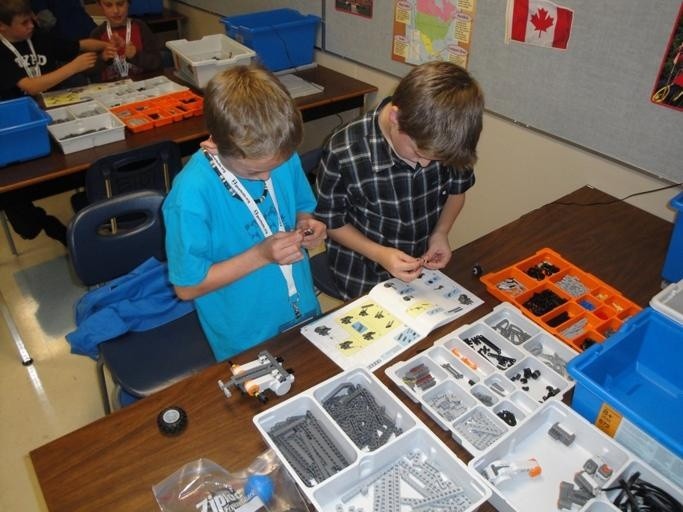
[44,215,67,246]
[71,193,88,213]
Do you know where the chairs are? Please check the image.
[65,189,215,419]
[68,139,186,236]
[295,147,352,312]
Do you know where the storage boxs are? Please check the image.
[218,8,322,72]
[126,1,164,17]
[566,307,683,494]
[648,277,683,327]
[164,33,256,90]
[658,191,683,282]
[0,95,53,167]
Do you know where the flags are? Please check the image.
[511,0,573,49]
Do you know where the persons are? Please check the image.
[0,0,126,248]
[83,0,161,83]
[161,64,329,362]
[310,60,485,300]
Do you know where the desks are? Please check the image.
[0,61,378,365]
[129,8,187,40]
[26,183,683,512]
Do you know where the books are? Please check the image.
[300,269,486,373]
